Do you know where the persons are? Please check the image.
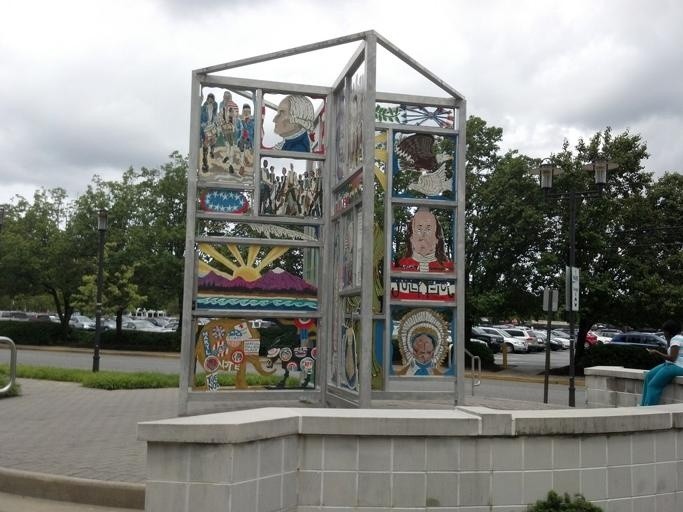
[640,319,683,406]
[199,89,254,175]
[272,94,316,152]
[399,208,452,272]
[260,160,323,218]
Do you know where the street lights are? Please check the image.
[525,151,627,407]
[87,205,113,372]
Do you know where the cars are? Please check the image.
[387,319,683,364]
[1,301,277,333]
